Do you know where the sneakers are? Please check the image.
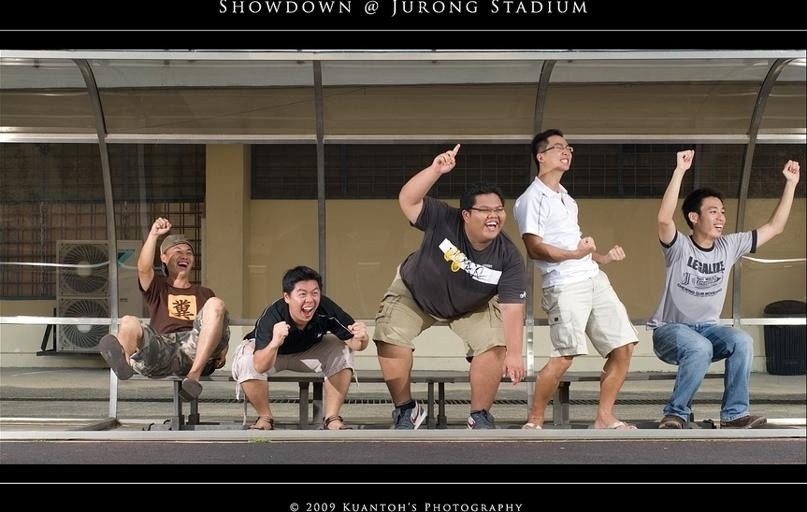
[657,416,689,429]
[721,416,767,429]
[467,409,495,429]
[389,401,427,430]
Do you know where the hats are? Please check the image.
[160,234,195,277]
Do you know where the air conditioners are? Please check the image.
[53,238,144,355]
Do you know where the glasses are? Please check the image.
[541,144,574,155]
[465,206,505,213]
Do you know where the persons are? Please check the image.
[373,143,528,429]
[232,266,369,430]
[512,130,644,429]
[97,217,231,402]
[646,149,800,430]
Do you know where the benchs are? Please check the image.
[121,368,733,432]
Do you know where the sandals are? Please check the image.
[522,422,542,430]
[178,378,202,402]
[323,415,351,431]
[588,422,639,430]
[249,417,274,430]
[98,334,134,380]
